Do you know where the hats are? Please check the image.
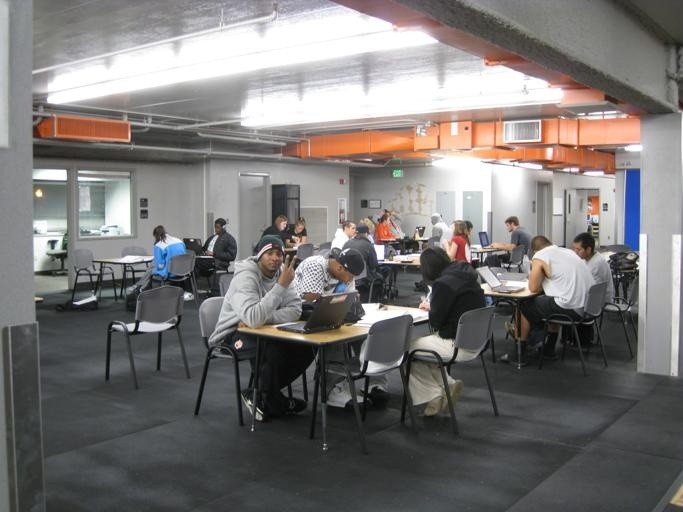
[331,247,364,276]
[254,234,286,260]
[356,223,370,235]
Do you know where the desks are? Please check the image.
[238,303,431,452]
[480,280,544,372]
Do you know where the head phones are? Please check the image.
[218,219,226,230]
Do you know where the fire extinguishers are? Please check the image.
[340,209,345,224]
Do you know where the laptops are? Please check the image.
[276,290,359,334]
[374,244,386,260]
[476,265,526,293]
[183,238,207,256]
[413,226,426,238]
[478,232,499,249]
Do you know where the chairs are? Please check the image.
[45,242,638,305]
[538,280,610,377]
[398,304,500,434]
[193,295,257,428]
[104,284,192,389]
[313,314,420,452]
[599,275,639,360]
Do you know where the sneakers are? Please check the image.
[368,380,388,395]
[423,398,441,417]
[286,398,307,412]
[505,320,527,350]
[326,388,373,410]
[241,389,264,422]
[441,380,464,412]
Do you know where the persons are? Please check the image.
[262,215,307,248]
[331,212,403,302]
[183,218,237,300]
[483,216,530,266]
[414,213,474,292]
[407,246,486,417]
[211,234,317,423]
[500,233,614,367]
[293,247,366,412]
[151,225,186,280]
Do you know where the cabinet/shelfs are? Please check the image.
[587,224,599,238]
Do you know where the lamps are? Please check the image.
[35,189,43,198]
